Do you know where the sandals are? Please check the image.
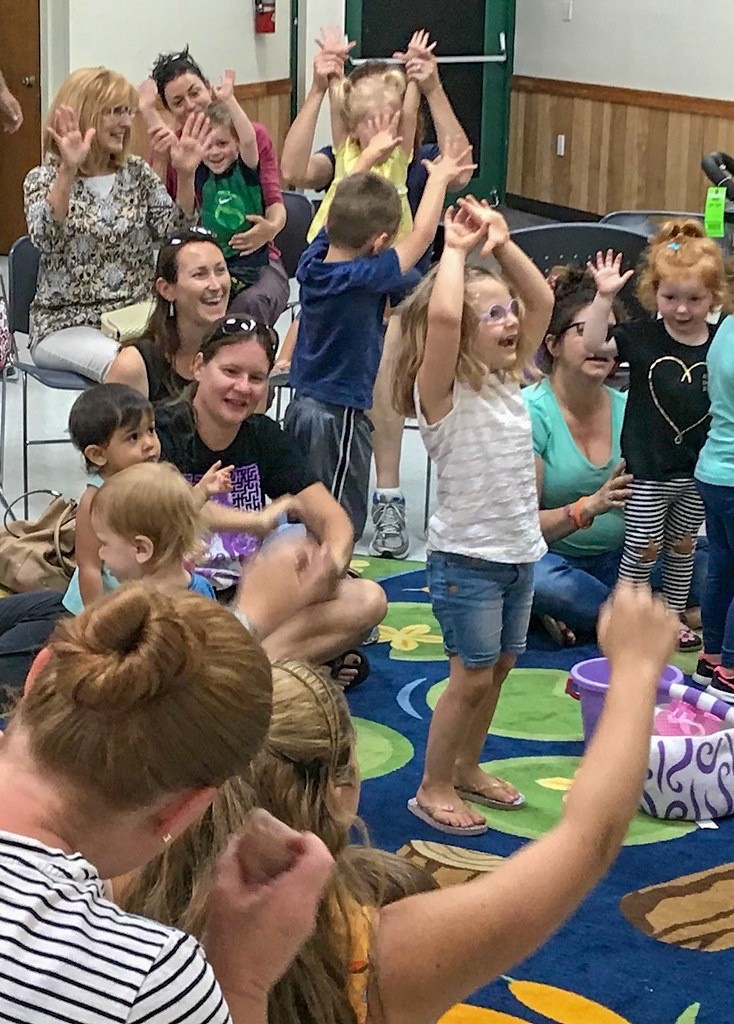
[320,647,370,692]
[531,608,577,648]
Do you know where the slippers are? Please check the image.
[407,796,489,836]
[452,777,525,810]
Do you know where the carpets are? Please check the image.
[343,554,734,1024]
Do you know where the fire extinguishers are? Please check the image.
[255,0,276,34]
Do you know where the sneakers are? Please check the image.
[370,490,410,559]
[692,652,722,686]
[705,666,734,704]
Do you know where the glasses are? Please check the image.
[558,322,615,341]
[161,226,216,249]
[202,318,278,373]
[99,104,136,120]
[152,52,201,77]
[476,298,520,326]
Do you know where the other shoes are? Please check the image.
[0,353,18,380]
[268,359,292,391]
[672,614,702,651]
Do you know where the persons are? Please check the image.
[89,463,217,603]
[99,537,678,1024]
[61,384,234,615]
[392,195,555,836]
[0,579,335,1024]
[0,30,734,689]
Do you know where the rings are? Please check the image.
[417,64,423,73]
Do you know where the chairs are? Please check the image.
[0,187,729,527]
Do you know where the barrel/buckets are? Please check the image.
[566,657,684,750]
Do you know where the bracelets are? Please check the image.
[574,496,594,530]
[563,504,581,530]
[232,607,259,636]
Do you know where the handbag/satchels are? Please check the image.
[103,299,158,343]
[0,489,80,596]
